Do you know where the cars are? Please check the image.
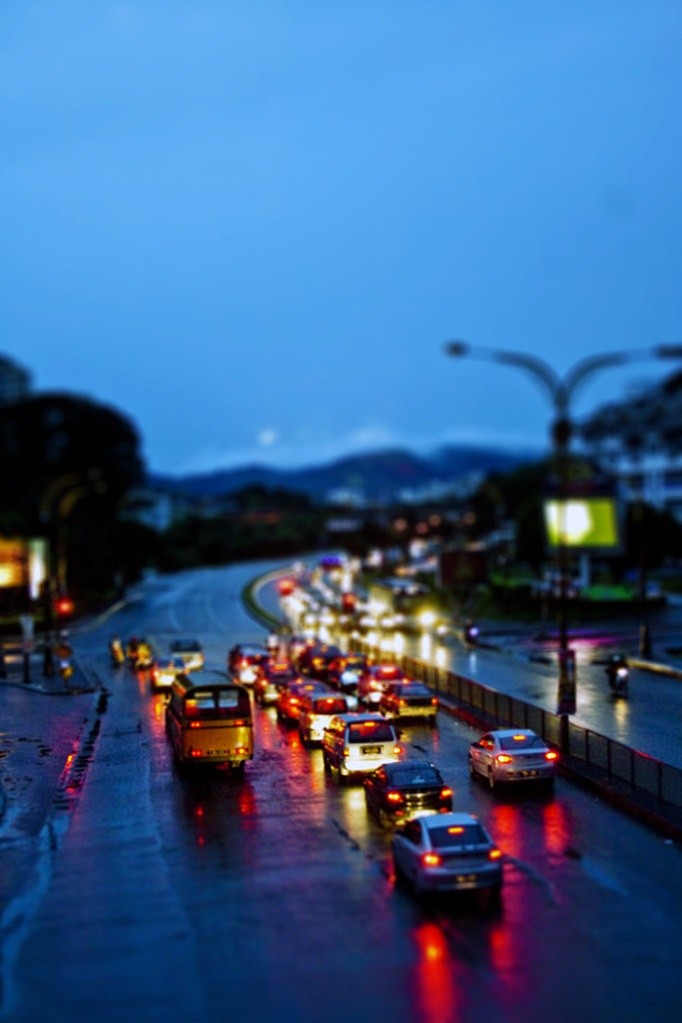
[279,682,326,721]
[280,552,456,650]
[379,684,441,722]
[469,726,559,789]
[150,636,372,705]
[390,810,502,898]
[365,760,454,828]
[359,664,409,702]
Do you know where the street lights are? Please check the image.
[449,341,682,783]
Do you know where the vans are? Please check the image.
[165,667,253,776]
[300,698,348,740]
[320,712,401,780]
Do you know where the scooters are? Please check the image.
[607,667,629,695]
